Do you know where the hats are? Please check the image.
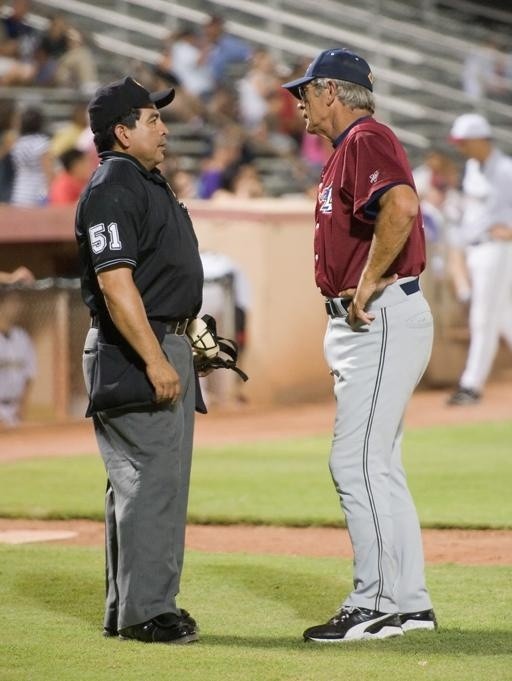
[447,114,492,144]
[280,46,375,99]
[86,76,177,134]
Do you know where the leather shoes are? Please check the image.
[103,611,195,639]
[119,613,196,648]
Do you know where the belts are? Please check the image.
[89,314,191,338]
[326,277,421,318]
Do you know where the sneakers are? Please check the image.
[395,608,438,635]
[444,385,479,410]
[301,607,404,646]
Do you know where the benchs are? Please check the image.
[1,35,324,199]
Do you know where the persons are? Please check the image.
[75,76,204,643]
[281,50,438,644]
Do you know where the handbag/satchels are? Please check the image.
[84,344,178,423]
